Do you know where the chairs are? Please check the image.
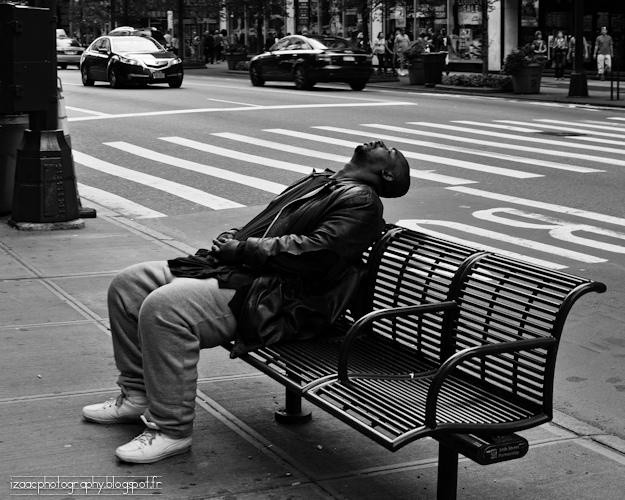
[221,222,605,500]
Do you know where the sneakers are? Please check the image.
[82,387,148,423]
[115,426,193,465]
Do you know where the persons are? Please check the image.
[354,28,456,74]
[531,24,615,81]
[165,29,280,64]
[83,140,411,463]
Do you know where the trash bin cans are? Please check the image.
[422,53,448,87]
[515,64,541,93]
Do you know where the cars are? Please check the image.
[249,35,374,92]
[57,26,181,88]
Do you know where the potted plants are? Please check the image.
[404,38,449,85]
[501,43,544,93]
